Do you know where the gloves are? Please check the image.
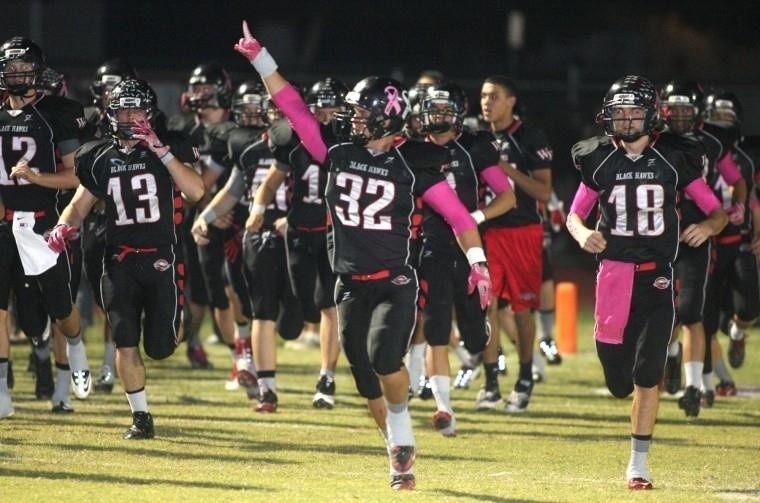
[225,231,246,262]
[233,20,279,79]
[132,117,171,159]
[726,202,745,225]
[115,245,158,261]
[48,222,81,254]
[548,201,566,232]
[464,248,492,310]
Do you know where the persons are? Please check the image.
[232,18,493,491]
[566,74,731,491]
[0,34,759,441]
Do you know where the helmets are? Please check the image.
[419,80,470,134]
[593,74,662,142]
[406,82,433,120]
[104,78,159,141]
[658,79,705,140]
[331,75,415,146]
[1,36,139,99]
[181,63,233,110]
[705,92,743,142]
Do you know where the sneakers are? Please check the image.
[664,318,747,417]
[408,364,481,437]
[389,452,416,492]
[385,410,416,472]
[0,348,114,419]
[311,374,336,409]
[186,342,260,399]
[121,411,155,439]
[253,383,278,413]
[625,451,654,489]
[476,337,561,413]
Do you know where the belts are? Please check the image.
[351,268,389,281]
[636,261,657,272]
[719,236,742,244]
[297,225,328,232]
[3,210,44,220]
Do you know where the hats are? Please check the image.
[231,78,356,131]
